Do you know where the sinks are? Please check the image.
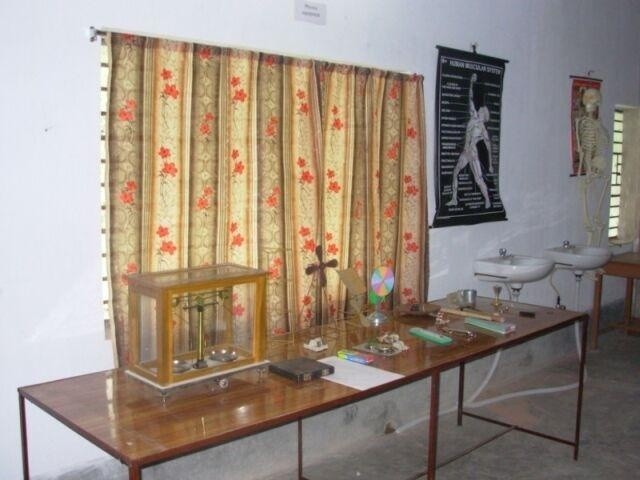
[543,246,611,269]
[473,255,555,281]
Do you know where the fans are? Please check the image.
[307,245,337,326]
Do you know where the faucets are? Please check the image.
[563,239,569,248]
[499,247,506,257]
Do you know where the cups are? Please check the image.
[448,288,477,307]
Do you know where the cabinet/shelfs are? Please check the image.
[125,262,274,402]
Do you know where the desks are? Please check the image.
[18,294,588,480]
[589,251,640,349]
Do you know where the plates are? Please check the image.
[210,348,238,361]
[172,360,192,373]
